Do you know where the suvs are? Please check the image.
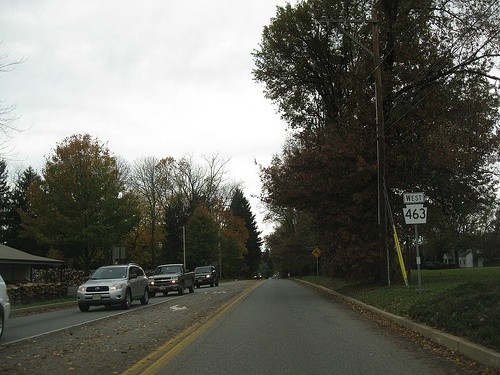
[77,263,150,312]
[194,266,218,288]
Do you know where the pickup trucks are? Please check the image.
[148,264,195,297]
